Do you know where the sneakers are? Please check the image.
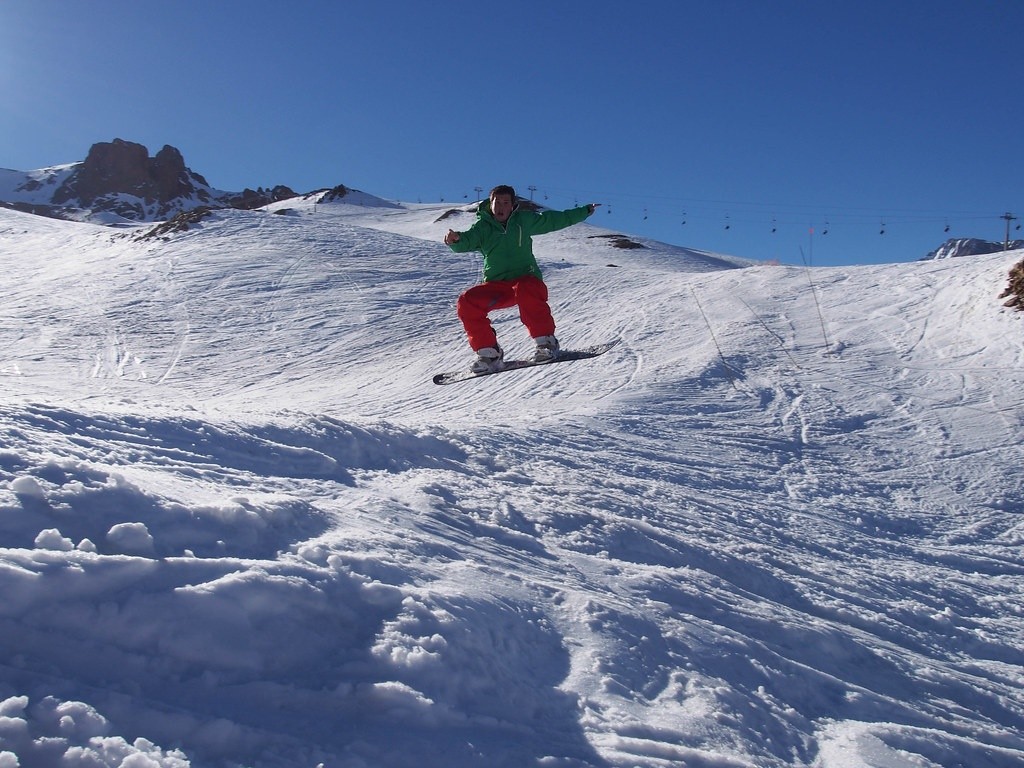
[534,335,560,364]
[470,343,504,375]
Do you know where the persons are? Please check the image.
[444,185,602,372]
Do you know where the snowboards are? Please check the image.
[432,338,621,386]
